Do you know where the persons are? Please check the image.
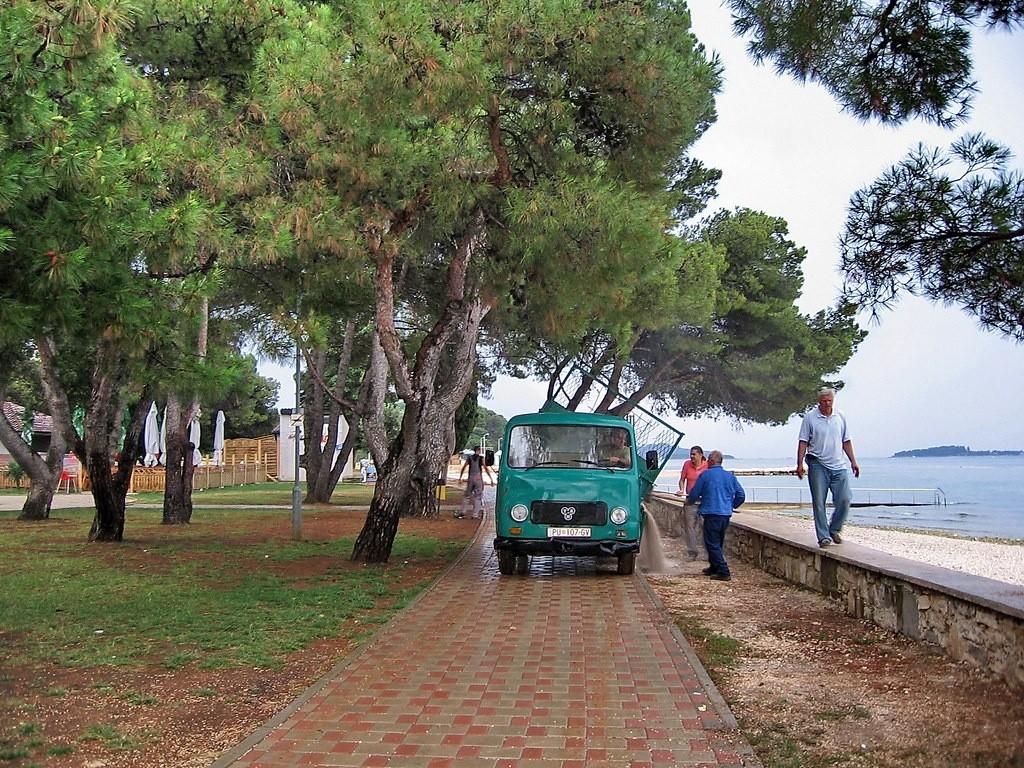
[684,451,745,580]
[796,386,859,548]
[676,446,708,562]
[458,446,494,520]
[597,428,631,468]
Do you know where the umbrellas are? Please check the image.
[189,409,202,466]
[159,407,166,466]
[143,401,159,467]
[213,411,226,467]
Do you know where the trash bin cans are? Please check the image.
[436,478,447,501]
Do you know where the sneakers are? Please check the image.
[702,566,720,575]
[710,572,730,581]
[819,538,830,548]
[830,531,841,544]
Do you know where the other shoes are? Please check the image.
[471,515,479,519]
[457,513,466,519]
[702,552,709,560]
[685,550,696,560]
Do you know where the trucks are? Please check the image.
[485,358,685,575]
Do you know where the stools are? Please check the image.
[56,471,77,493]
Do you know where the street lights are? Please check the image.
[497,438,502,450]
[481,433,490,454]
